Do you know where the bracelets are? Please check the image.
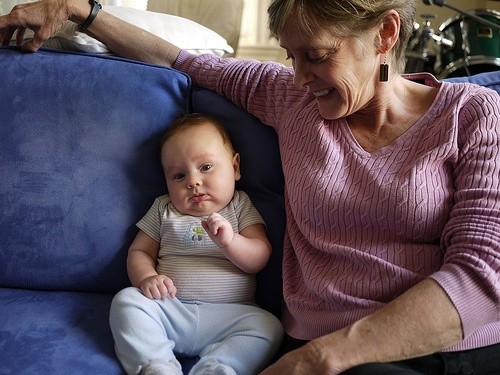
[76,0,102,33]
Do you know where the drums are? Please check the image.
[436,8,500,79]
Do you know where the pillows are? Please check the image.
[9,5,234,58]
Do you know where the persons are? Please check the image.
[0,0,500,375]
[107,110,285,375]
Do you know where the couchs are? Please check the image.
[0,47,500,375]
